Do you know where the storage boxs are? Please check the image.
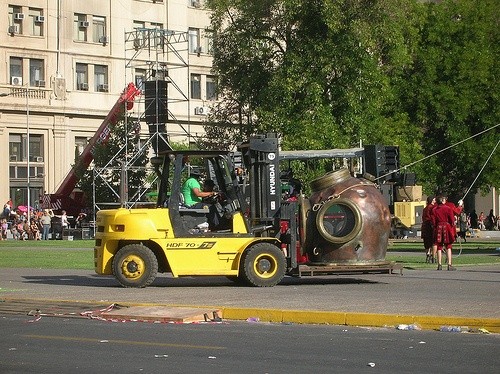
[62,227,90,240]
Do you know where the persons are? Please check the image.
[421,193,500,264]
[0,200,88,241]
[431,194,456,271]
[180,170,221,231]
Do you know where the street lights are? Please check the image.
[0,84,35,245]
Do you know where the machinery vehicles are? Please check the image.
[40,82,142,239]
[94,133,400,288]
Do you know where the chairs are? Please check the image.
[177,193,209,214]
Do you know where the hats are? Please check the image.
[191,168,204,176]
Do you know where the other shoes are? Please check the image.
[448,266,456,270]
[437,265,442,270]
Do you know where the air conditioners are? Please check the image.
[11,77,22,85]
[99,84,108,91]
[15,13,25,19]
[81,83,88,89]
[35,16,45,23]
[196,107,207,115]
[79,21,90,27]
[36,80,46,87]
[34,156,44,162]
[8,25,19,33]
